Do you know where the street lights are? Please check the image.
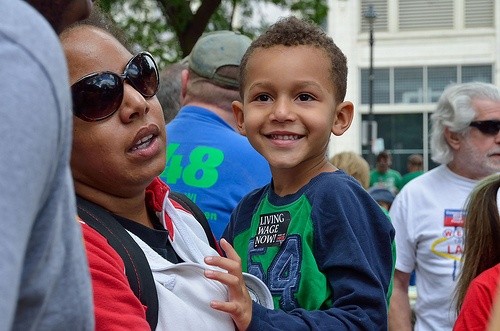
[364,4,377,175]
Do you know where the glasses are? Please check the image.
[71,52,165,122]
[468,120,500,135]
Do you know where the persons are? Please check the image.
[206,13,396,331]
[448,170,500,331]
[389,82,500,331]
[150,29,274,241]
[59,1,238,331]
[1,0,94,331]
[327,150,427,286]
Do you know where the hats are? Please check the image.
[188,30,270,88]
[370,189,393,203]
